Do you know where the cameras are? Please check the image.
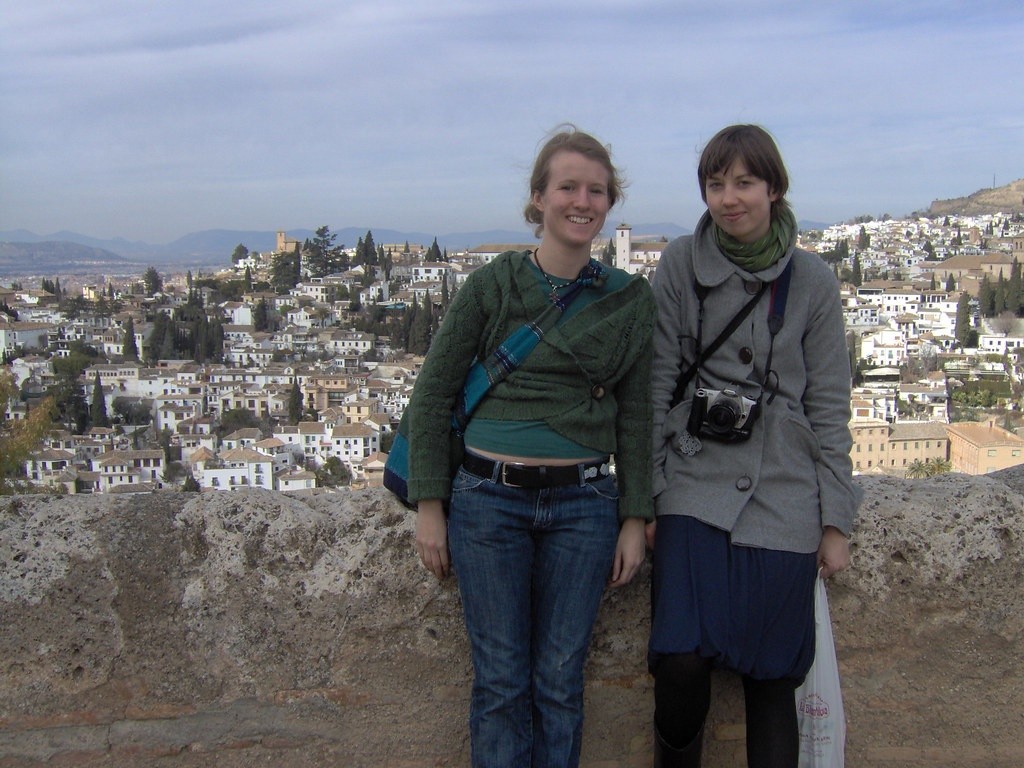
[687,387,761,444]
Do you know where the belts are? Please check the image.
[462,451,610,489]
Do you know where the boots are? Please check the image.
[654,654,711,768]
[742,678,799,768]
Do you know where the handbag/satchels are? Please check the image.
[382,360,478,514]
[792,567,847,768]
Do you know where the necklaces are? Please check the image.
[534,248,591,311]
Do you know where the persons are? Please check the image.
[653,124,858,768]
[409,124,657,768]
